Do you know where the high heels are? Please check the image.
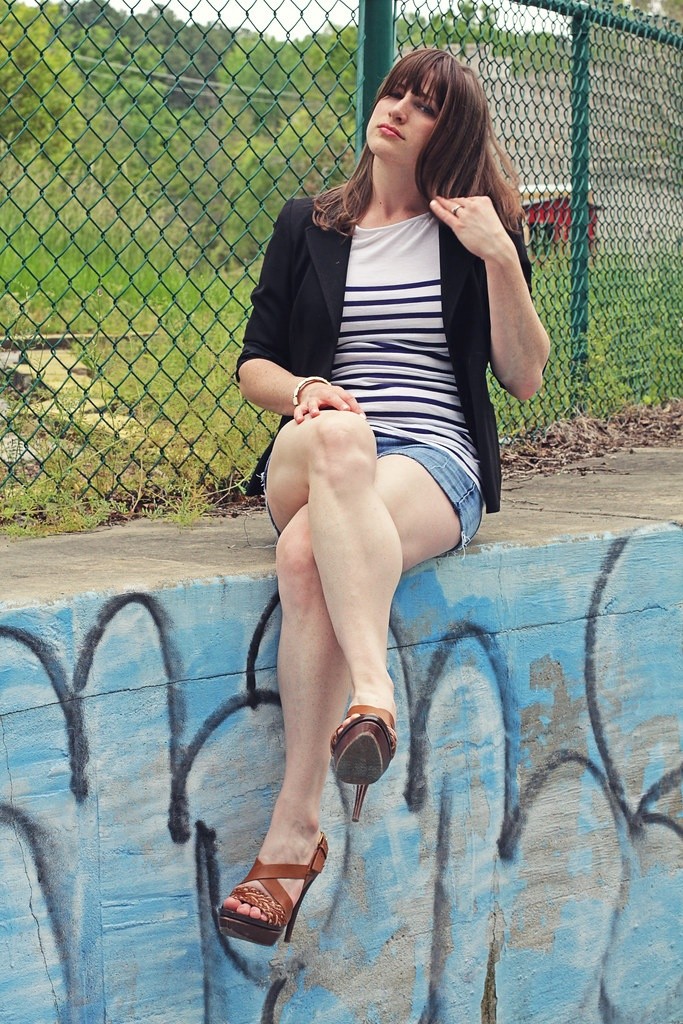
[330,704,396,821]
[218,831,329,948]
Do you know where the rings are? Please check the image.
[451,205,463,215]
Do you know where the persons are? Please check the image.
[217,49,551,947]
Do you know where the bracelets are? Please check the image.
[292,376,332,406]
